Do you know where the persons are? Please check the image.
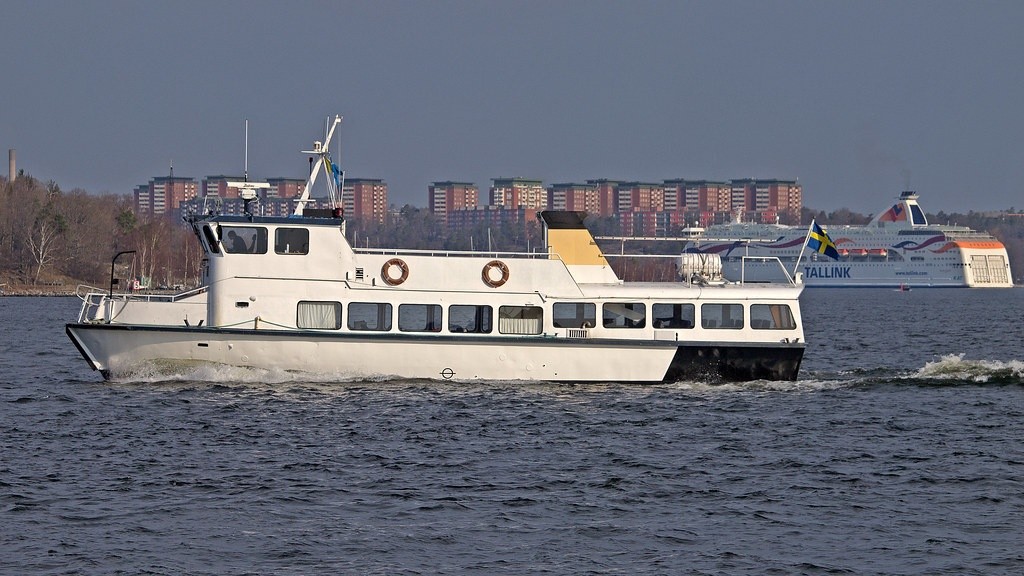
[228,230,247,253]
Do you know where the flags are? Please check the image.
[323,150,340,187]
[807,222,840,262]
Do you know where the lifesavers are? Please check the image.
[382,258,410,286]
[482,259,511,288]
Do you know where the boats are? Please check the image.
[670,190,1015,290]
[848,249,868,258]
[868,249,887,258]
[836,249,848,258]
[64,115,808,384]
